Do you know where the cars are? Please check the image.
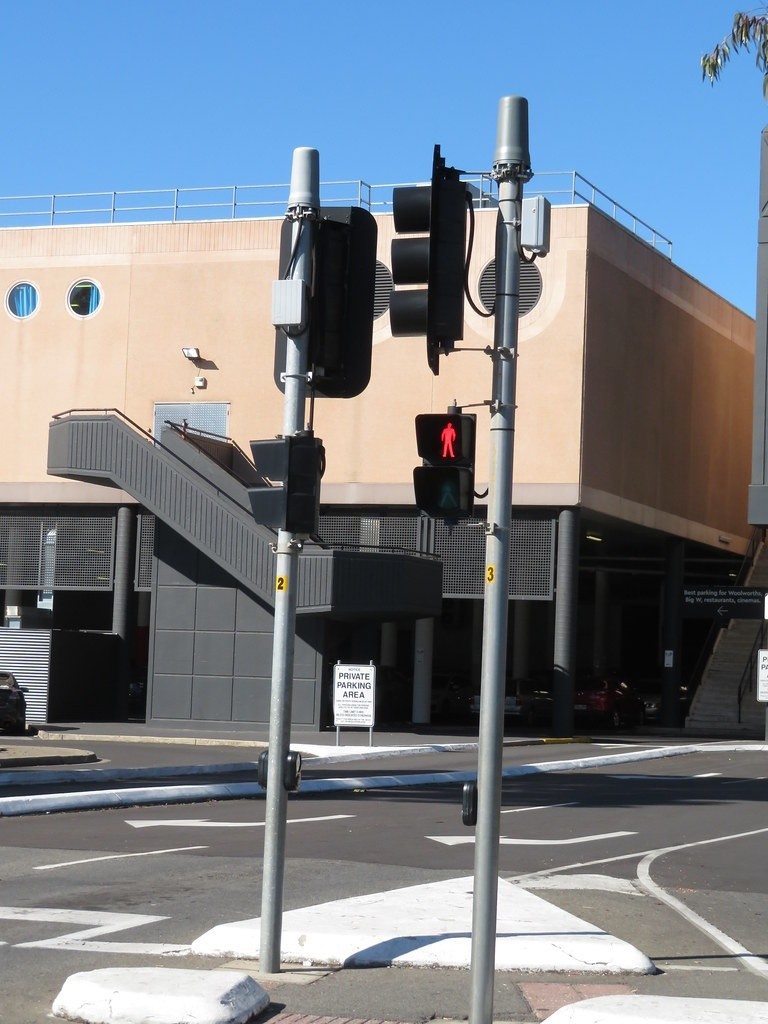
[637,690,666,723]
[468,677,556,723]
[127,674,149,707]
[0,671,28,735]
[570,676,636,731]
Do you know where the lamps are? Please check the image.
[182,346,202,362]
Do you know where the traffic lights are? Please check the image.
[413,412,479,521]
[389,141,467,375]
[246,434,325,533]
